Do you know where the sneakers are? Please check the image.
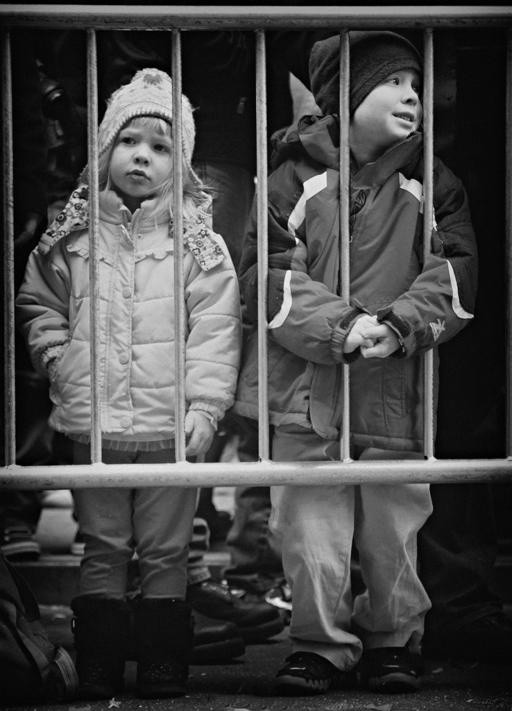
[359,646,424,691]
[273,652,349,693]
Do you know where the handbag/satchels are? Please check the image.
[0,551,81,705]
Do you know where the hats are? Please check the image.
[76,67,204,198]
[308,30,423,142]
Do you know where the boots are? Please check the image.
[133,592,193,698]
[71,596,129,700]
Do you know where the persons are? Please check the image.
[19,67,241,699]
[241,29,480,696]
